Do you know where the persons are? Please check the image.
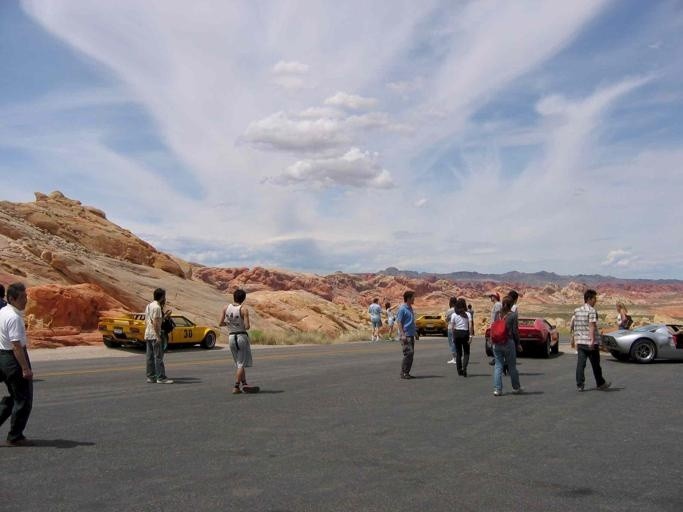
[219,289,261,394]
[368,290,634,396]
[159,298,172,379]
[0,281,35,446]
[0,283,9,383]
[145,288,176,383]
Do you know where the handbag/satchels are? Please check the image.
[163,317,174,334]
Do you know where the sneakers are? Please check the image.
[233,385,259,393]
[6,439,35,447]
[400,358,612,397]
[148,376,174,384]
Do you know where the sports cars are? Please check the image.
[97,311,220,350]
[600,324,682,365]
[415,314,448,336]
[484,317,559,357]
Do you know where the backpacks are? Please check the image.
[490,312,512,346]
